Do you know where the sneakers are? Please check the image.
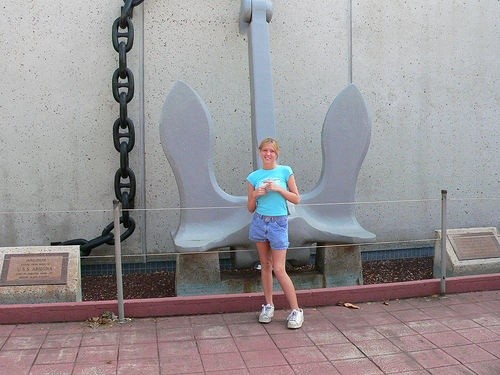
[287,308,304,329]
[259,304,274,323]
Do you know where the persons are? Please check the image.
[245,138,307,329]
